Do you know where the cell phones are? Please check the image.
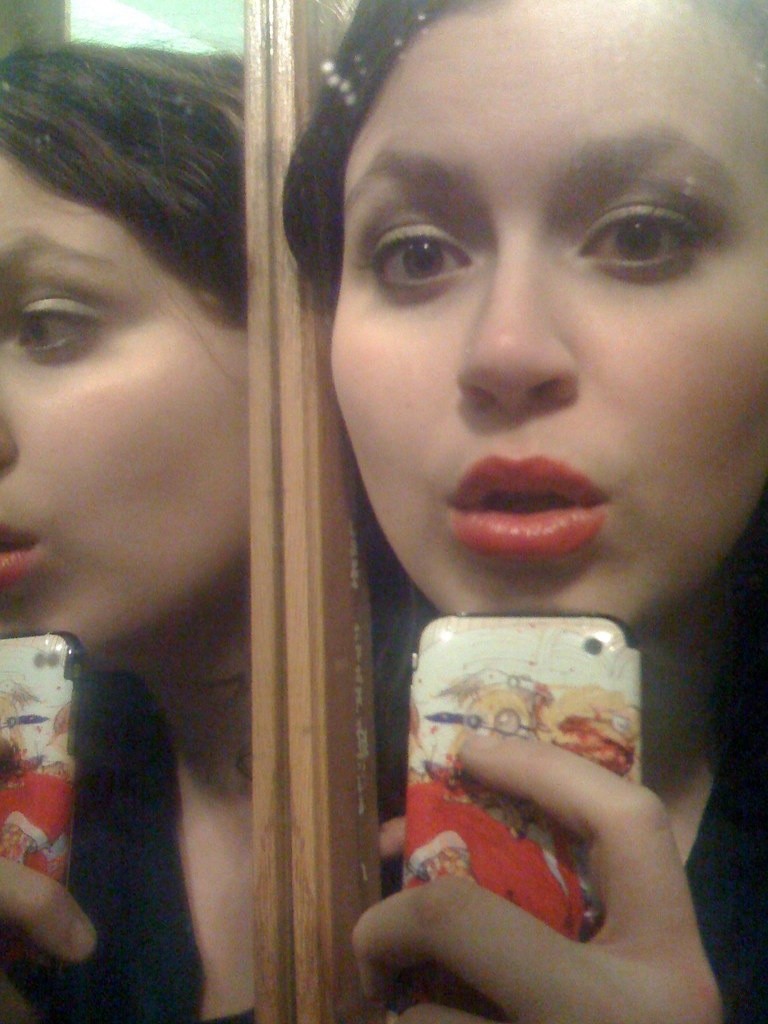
[409,615,645,1014]
[2,629,91,970]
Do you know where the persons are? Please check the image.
[0,39,248,1024]
[281,0,768,1024]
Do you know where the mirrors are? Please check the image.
[0,1,397,1024]
